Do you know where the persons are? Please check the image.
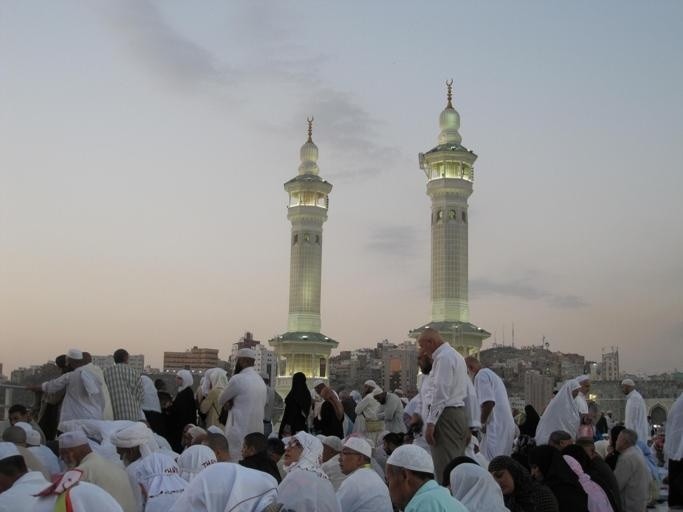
[0,326,682,510]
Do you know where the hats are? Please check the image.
[0,441,21,462]
[386,443,435,474]
[236,348,256,361]
[365,380,383,396]
[322,435,373,459]
[576,375,589,383]
[622,379,635,386]
[59,430,87,449]
[66,348,83,360]
[313,380,324,388]
[207,425,224,436]
